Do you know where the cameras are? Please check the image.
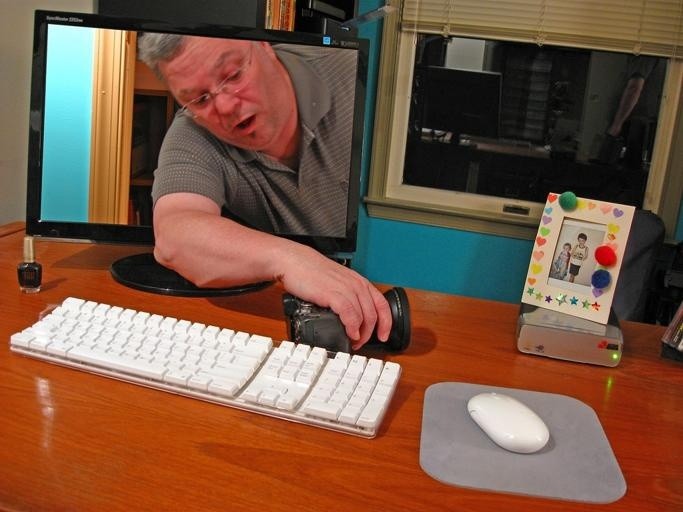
[276,287,412,355]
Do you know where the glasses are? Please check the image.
[179,43,254,119]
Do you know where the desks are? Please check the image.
[0,222,683,512]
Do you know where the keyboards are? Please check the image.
[8,297,403,441]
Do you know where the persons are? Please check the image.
[135,31,393,350]
[549,242,572,280]
[593,53,669,173]
[567,232,590,284]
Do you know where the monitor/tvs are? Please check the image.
[416,64,504,149]
[23,6,370,297]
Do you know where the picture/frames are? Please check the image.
[521,193,635,325]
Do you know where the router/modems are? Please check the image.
[516,300,624,368]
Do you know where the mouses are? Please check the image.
[466,390,551,456]
